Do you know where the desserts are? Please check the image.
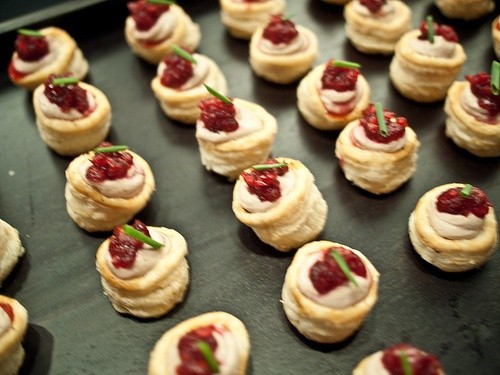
[0,0,500,375]
